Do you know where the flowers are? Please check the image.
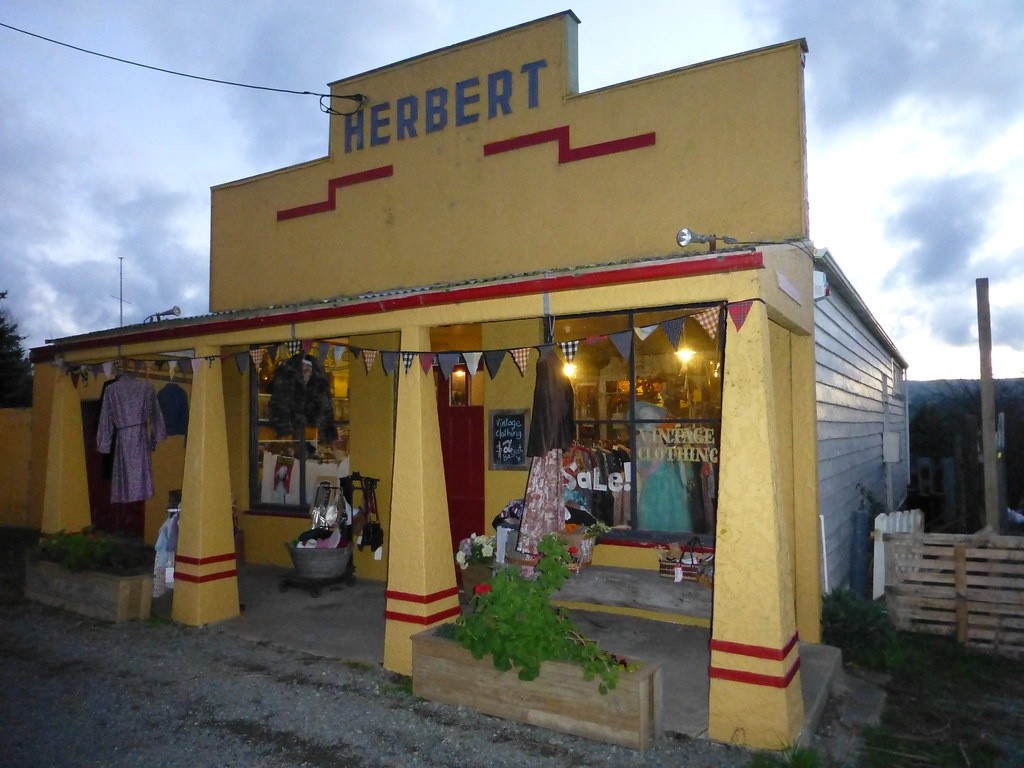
[23,524,156,577]
[456,532,498,571]
[435,533,641,697]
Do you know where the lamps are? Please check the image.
[673,324,697,364]
[143,306,182,324]
[677,228,718,252]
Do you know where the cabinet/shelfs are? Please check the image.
[256,391,350,467]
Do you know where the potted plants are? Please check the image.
[573,522,611,567]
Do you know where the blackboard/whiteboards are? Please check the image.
[487,407,533,472]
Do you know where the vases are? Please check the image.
[24,561,154,623]
[409,624,663,752]
[460,565,493,601]
